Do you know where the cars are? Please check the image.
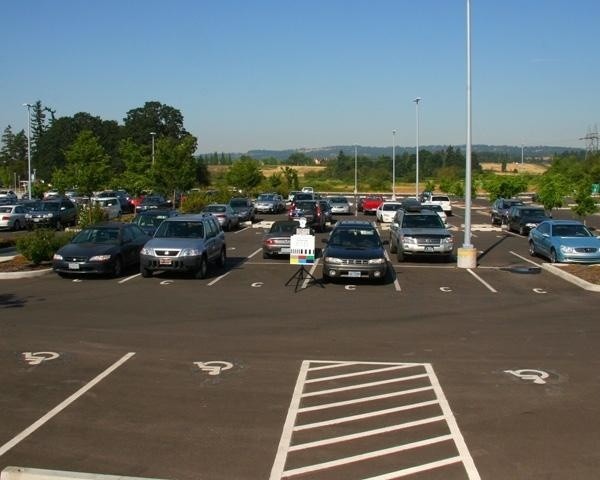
[328,196,350,214]
[421,202,447,224]
[136,195,173,214]
[201,204,240,231]
[507,205,547,236]
[0,203,24,231]
[376,201,401,223]
[229,198,254,222]
[321,220,390,285]
[254,192,285,214]
[52,222,150,279]
[528,219,599,264]
[0,189,18,201]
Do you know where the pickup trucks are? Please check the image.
[362,194,384,215]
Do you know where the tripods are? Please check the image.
[284,267,325,293]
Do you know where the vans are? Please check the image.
[289,192,331,226]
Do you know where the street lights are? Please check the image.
[412,96,422,201]
[391,128,396,201]
[23,102,37,201]
[150,132,157,163]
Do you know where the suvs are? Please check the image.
[489,198,524,228]
[429,193,452,217]
[140,214,227,280]
[91,197,122,220]
[389,206,455,260]
[24,197,75,228]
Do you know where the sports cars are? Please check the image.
[263,220,301,258]
